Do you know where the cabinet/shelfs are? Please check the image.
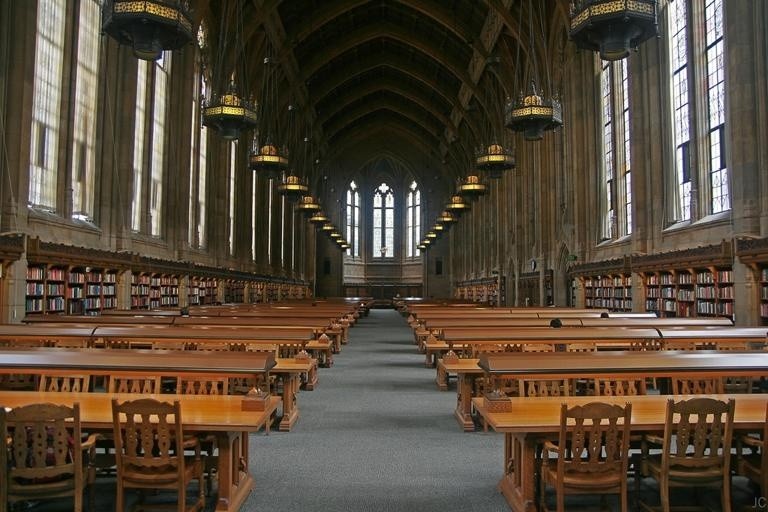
[13,257,311,314]
[175,371,231,397]
[451,264,735,321]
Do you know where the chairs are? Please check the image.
[663,339,699,352]
[103,333,133,352]
[670,371,725,395]
[111,395,206,512]
[474,344,505,357]
[518,378,568,396]
[244,340,281,361]
[593,372,647,396]
[521,341,556,354]
[565,341,598,354]
[38,368,92,394]
[714,341,752,394]
[195,339,231,353]
[732,403,768,509]
[633,394,736,512]
[0,400,103,512]
[0,335,44,391]
[536,398,633,512]
[630,338,666,353]
[107,369,162,395]
[47,337,87,351]
[148,339,188,354]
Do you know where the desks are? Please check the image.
[470,394,767,512]
[392,299,440,354]
[0,389,283,511]
[306,333,335,369]
[324,294,376,355]
[421,332,468,370]
[272,352,319,433]
[434,355,483,433]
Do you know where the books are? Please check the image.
[87,285,100,295]
[26,299,42,311]
[718,302,732,315]
[677,288,695,301]
[646,287,659,297]
[27,267,43,280]
[583,277,632,312]
[189,277,217,305]
[663,299,676,311]
[68,299,84,314]
[760,269,768,317]
[679,273,691,284]
[660,274,672,284]
[717,271,733,282]
[86,311,99,316]
[47,297,65,310]
[718,286,733,298]
[67,287,83,299]
[645,298,659,310]
[104,296,118,308]
[677,302,690,317]
[87,271,101,281]
[130,273,179,310]
[660,287,676,298]
[696,272,714,283]
[85,298,100,307]
[68,272,84,283]
[103,286,114,295]
[47,284,65,295]
[646,275,658,285]
[697,300,716,314]
[48,269,64,280]
[103,273,115,282]
[695,285,714,298]
[26,282,44,295]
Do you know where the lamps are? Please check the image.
[198,68,258,141]
[432,223,446,231]
[309,211,328,225]
[93,1,198,64]
[297,193,322,215]
[566,1,662,65]
[446,195,472,217]
[460,175,486,204]
[249,115,289,182]
[421,239,432,245]
[417,243,429,252]
[335,237,347,245]
[476,145,515,181]
[321,222,335,231]
[503,0,563,142]
[340,243,352,252]
[425,232,438,239]
[330,231,342,239]
[436,209,459,228]
[277,168,309,203]
